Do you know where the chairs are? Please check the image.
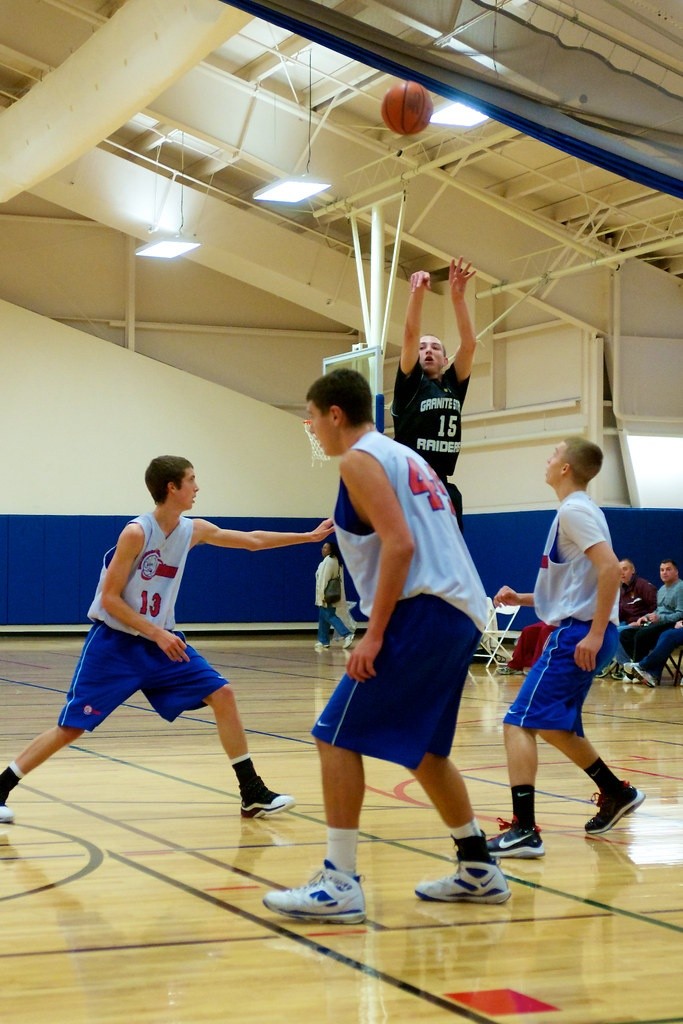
[659,643,683,689]
[470,603,522,668]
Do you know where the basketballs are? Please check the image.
[380,80,434,136]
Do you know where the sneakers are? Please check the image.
[342,634,355,649]
[622,675,632,684]
[239,776,297,818]
[585,780,646,834]
[595,660,617,678]
[623,663,642,671]
[263,859,367,924]
[632,677,641,684]
[486,815,545,858]
[415,850,512,904]
[611,668,625,679]
[496,666,524,675]
[315,642,331,648]
[0,806,14,822]
[632,667,656,688]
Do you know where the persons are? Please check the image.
[392,255,477,524]
[487,436,644,857]
[508,623,557,675]
[621,562,683,683]
[623,621,683,687]
[315,543,355,647]
[0,454,335,820]
[595,558,656,679]
[263,369,511,922]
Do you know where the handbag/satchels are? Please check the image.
[324,565,342,603]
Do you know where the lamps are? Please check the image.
[132,131,203,260]
[429,0,499,130]
[249,47,334,205]
